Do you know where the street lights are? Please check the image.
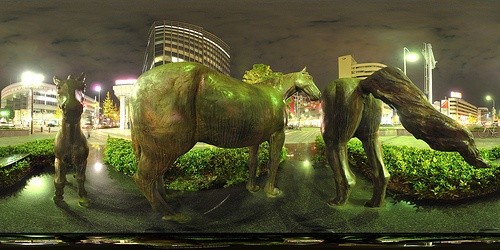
[94,86,101,105]
[485,96,496,108]
[402,46,419,75]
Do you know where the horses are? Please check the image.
[49,71,93,210]
[319,63,493,209]
[127,59,324,226]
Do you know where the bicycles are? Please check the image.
[477,121,500,139]
